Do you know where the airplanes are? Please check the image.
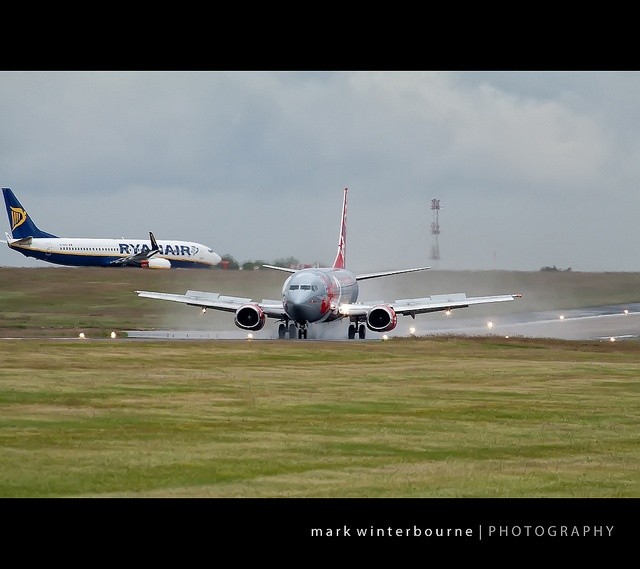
[0,188,222,269]
[134,188,522,339]
